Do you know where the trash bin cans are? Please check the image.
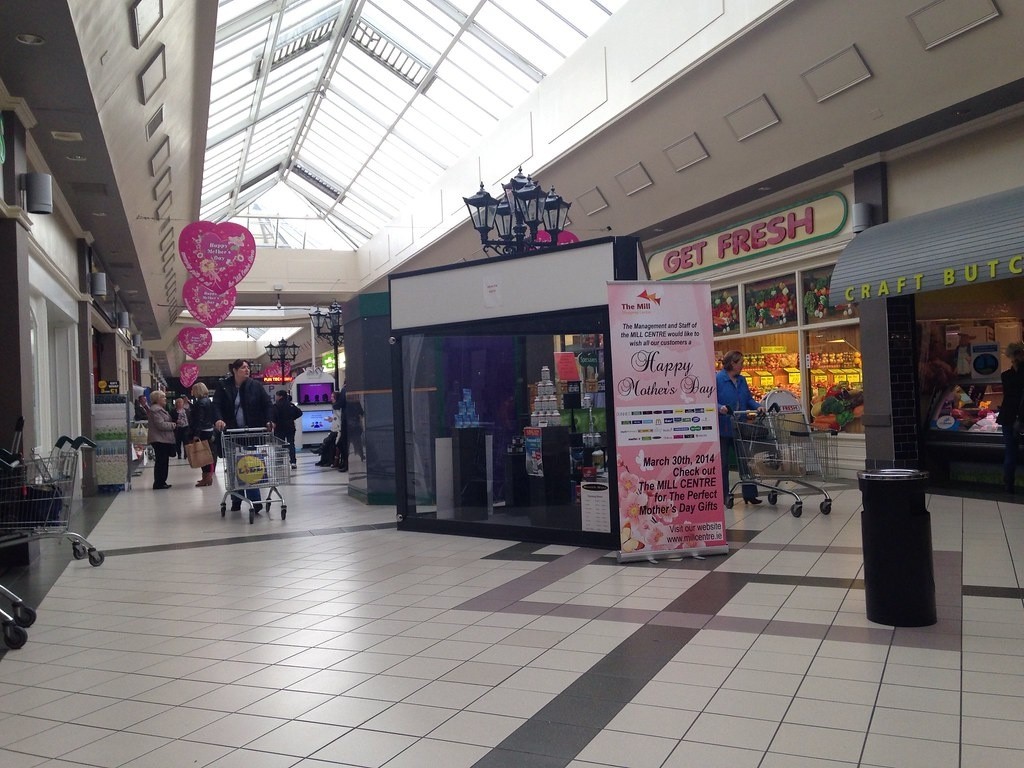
[857,469,938,626]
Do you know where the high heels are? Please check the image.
[310,448,320,456]
[195,473,213,487]
[742,496,762,504]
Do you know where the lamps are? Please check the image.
[20,170,53,215]
[118,311,128,329]
[133,334,140,346]
[151,358,168,386]
[90,270,107,296]
[277,294,281,309]
[139,347,145,359]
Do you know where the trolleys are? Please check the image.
[0,585,37,650]
[0,416,105,566]
[726,402,838,518]
[220,426,291,525]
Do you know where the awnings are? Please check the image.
[827,183,1024,307]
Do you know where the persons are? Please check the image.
[137,383,220,490]
[273,390,303,469]
[310,378,347,472]
[716,351,766,504]
[347,394,367,462]
[999,342,1024,495]
[212,359,276,513]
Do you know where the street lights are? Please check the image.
[265,337,300,385]
[309,299,344,401]
[462,166,572,430]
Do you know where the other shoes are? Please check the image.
[255,503,262,514]
[153,482,171,489]
[231,504,240,511]
[315,460,348,472]
[1005,484,1015,493]
[178,456,181,459]
[291,463,297,469]
[184,456,187,459]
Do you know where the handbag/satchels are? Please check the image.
[169,446,176,457]
[130,423,149,444]
[210,428,225,458]
[184,437,214,469]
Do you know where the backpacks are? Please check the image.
[273,397,293,431]
